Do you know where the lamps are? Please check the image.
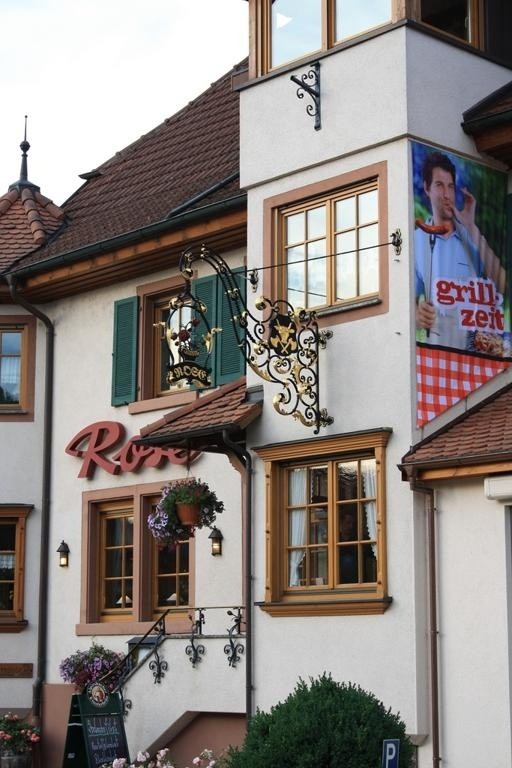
[56,539,71,567]
[208,522,222,555]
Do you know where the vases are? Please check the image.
[0,749,31,767]
[174,500,201,524]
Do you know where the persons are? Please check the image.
[339,511,374,584]
[408,149,507,354]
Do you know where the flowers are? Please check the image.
[100,746,219,768]
[0,710,42,755]
[147,478,225,544]
[59,644,128,694]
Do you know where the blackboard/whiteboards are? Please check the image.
[78,693,130,768]
